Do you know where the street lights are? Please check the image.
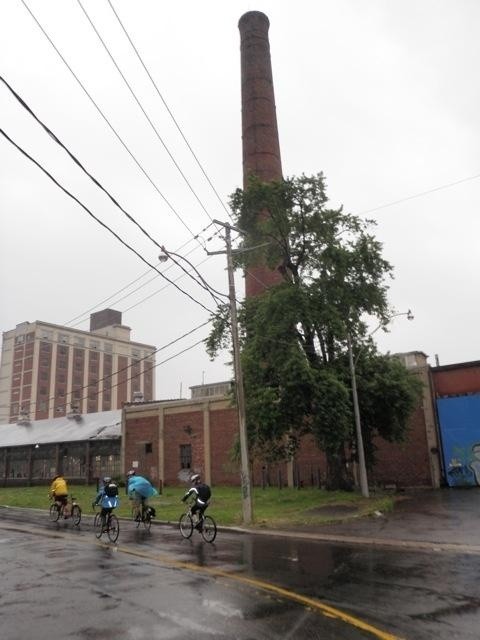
[155,247,253,527]
[351,309,416,498]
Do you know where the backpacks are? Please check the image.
[104,483,118,497]
[194,483,211,504]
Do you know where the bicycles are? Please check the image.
[178,500,217,544]
[129,496,153,531]
[47,489,82,526]
[92,502,120,543]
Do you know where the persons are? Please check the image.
[47,472,68,520]
[180,474,211,533]
[94,476,120,532]
[126,470,148,521]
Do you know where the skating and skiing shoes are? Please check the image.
[103,525,109,532]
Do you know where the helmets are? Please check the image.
[127,470,135,476]
[103,476,112,483]
[191,474,199,482]
[52,475,59,481]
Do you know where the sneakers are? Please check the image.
[194,520,200,528]
[56,505,62,511]
